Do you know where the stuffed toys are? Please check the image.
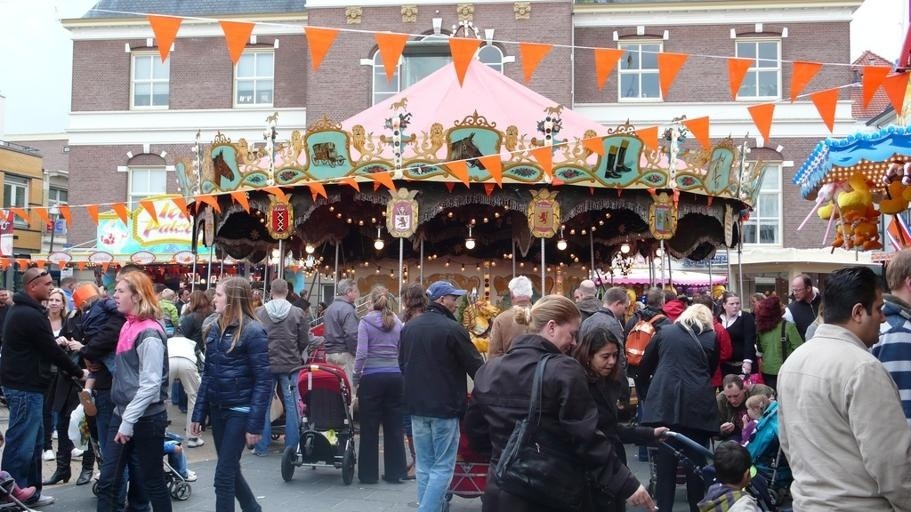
[817,162,911,251]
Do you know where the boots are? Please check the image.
[42,452,71,484]
[75,447,96,487]
[9,485,36,501]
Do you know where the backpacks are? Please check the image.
[625,313,665,366]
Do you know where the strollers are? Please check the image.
[281,364,356,485]
[0,478,38,512]
[71,377,191,501]
[443,393,490,504]
[740,401,792,505]
[660,431,779,512]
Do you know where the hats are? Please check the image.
[426,280,470,303]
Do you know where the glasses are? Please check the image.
[791,288,810,293]
[23,269,52,284]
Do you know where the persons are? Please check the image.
[180,292,215,352]
[0,432,36,508]
[159,289,181,336]
[572,287,631,414]
[110,271,173,512]
[152,283,168,295]
[624,288,671,461]
[401,285,427,321]
[0,290,14,316]
[715,292,755,377]
[79,264,151,511]
[578,279,603,322]
[251,279,309,456]
[1,266,89,511]
[688,295,731,394]
[697,442,762,511]
[573,289,585,307]
[315,302,326,317]
[488,277,533,359]
[323,279,360,433]
[42,280,99,485]
[660,291,685,320]
[776,267,909,511]
[789,274,820,343]
[165,333,205,448]
[867,247,911,421]
[636,304,719,512]
[163,432,197,481]
[398,282,485,511]
[191,277,273,511]
[571,328,669,512]
[352,286,407,483]
[175,288,190,318]
[293,290,310,309]
[463,294,653,511]
[753,295,803,390]
[741,395,770,445]
[287,282,299,303]
[717,374,777,443]
[54,276,78,311]
[72,281,128,417]
[42,288,84,461]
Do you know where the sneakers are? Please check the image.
[172,469,197,481]
[187,439,205,447]
[26,494,55,506]
